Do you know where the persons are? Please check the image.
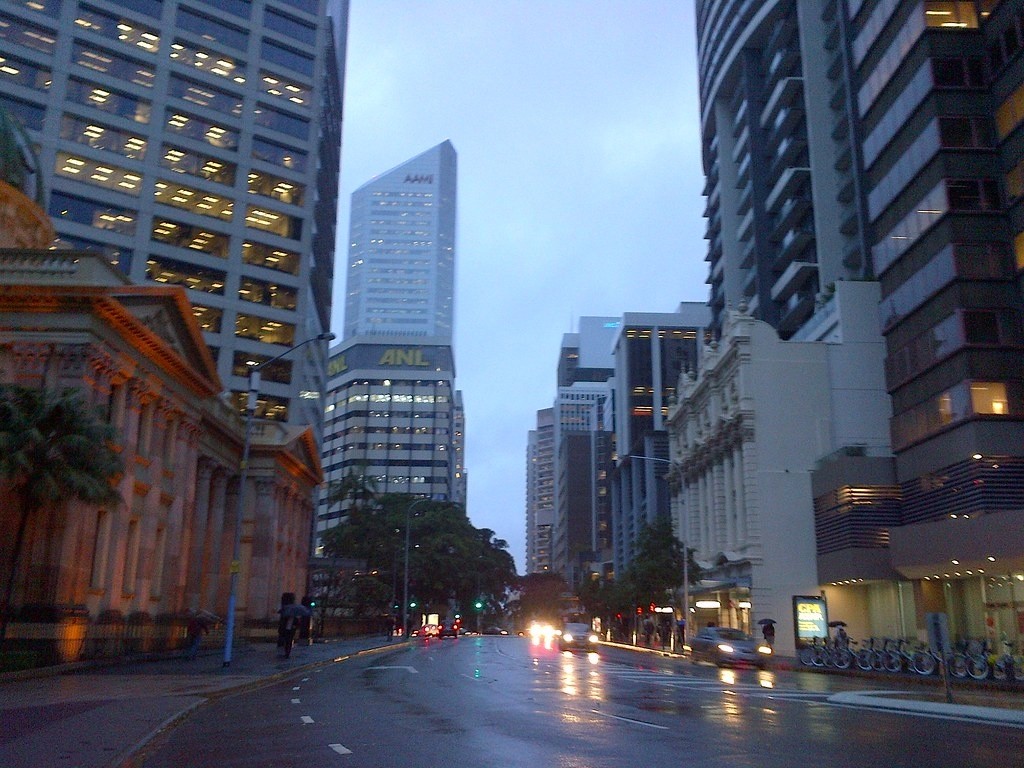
[838,629,850,642]
[644,620,672,650]
[676,625,686,650]
[275,615,298,659]
[186,610,210,661]
[762,623,774,646]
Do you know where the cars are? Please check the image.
[481,625,511,636]
[409,616,478,639]
[524,618,600,652]
[690,626,766,671]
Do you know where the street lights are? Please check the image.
[617,452,691,655]
[220,331,338,671]
[401,499,440,641]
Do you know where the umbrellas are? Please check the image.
[828,621,846,627]
[277,603,313,616]
[757,618,777,624]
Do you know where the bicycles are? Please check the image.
[799,629,1024,685]
